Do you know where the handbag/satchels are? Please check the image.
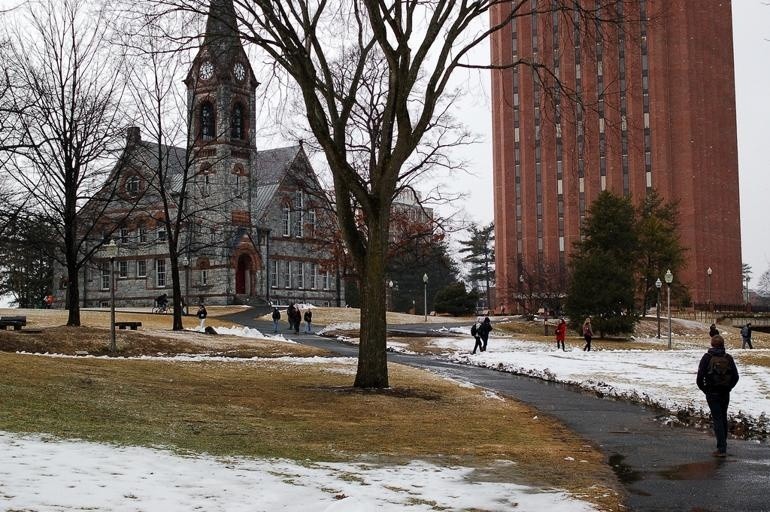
[583,324,592,336]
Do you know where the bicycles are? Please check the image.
[151,301,174,314]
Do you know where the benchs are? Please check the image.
[115,322,142,330]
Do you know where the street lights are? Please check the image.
[664,269,674,349]
[707,267,713,302]
[105,239,119,357]
[654,277,663,339]
[422,273,429,323]
[746,275,751,304]
[389,280,394,312]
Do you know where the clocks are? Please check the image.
[233,61,246,83]
[198,61,215,81]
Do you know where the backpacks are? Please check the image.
[478,324,486,335]
[705,352,732,388]
[742,326,748,337]
[471,324,477,336]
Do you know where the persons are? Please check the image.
[34,294,56,308]
[473,317,484,354]
[271,307,281,333]
[582,317,594,348]
[710,323,720,338]
[180,294,186,316]
[478,317,492,351]
[157,293,169,311]
[196,305,207,328]
[556,319,566,351]
[287,302,301,334]
[583,322,593,352]
[304,308,312,334]
[696,334,738,457]
[741,322,755,349]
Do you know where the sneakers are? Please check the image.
[479,347,486,352]
[473,351,476,354]
[712,451,725,456]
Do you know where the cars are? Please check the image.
[429,310,438,316]
[529,312,554,319]
